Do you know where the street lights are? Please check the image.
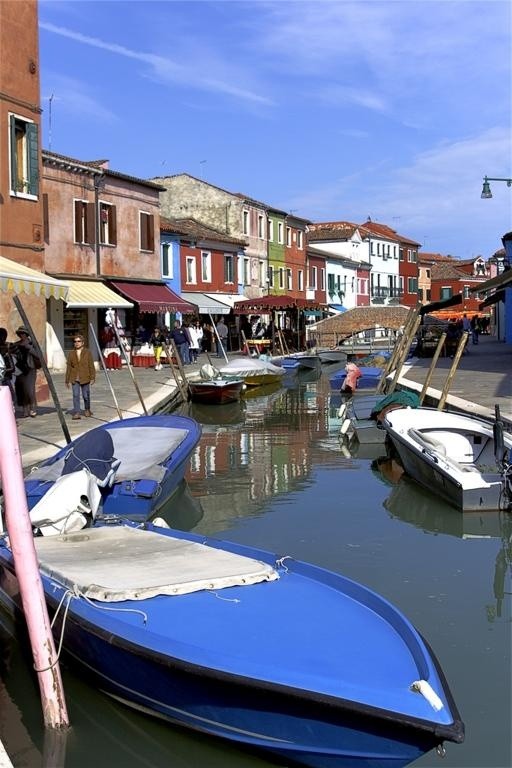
[486,257,501,343]
[480,176,512,199]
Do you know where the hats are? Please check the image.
[15,325,29,335]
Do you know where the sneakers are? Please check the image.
[30,410,37,417]
[19,414,28,418]
[73,412,81,419]
[85,409,91,417]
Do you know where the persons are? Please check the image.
[13,325,41,417]
[0,328,20,417]
[65,335,96,419]
[149,321,204,371]
[462,313,490,345]
[216,317,228,358]
[239,318,277,354]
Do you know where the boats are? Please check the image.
[339,391,402,444]
[383,405,510,513]
[329,350,391,389]
[146,476,205,531]
[245,381,284,399]
[1,514,469,765]
[218,358,288,386]
[321,361,348,372]
[381,472,511,620]
[1,413,203,522]
[284,369,323,383]
[318,350,349,364]
[190,400,248,425]
[281,354,321,377]
[345,443,388,459]
[189,375,246,406]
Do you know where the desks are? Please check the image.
[160,351,177,364]
[102,348,121,371]
[131,351,156,368]
